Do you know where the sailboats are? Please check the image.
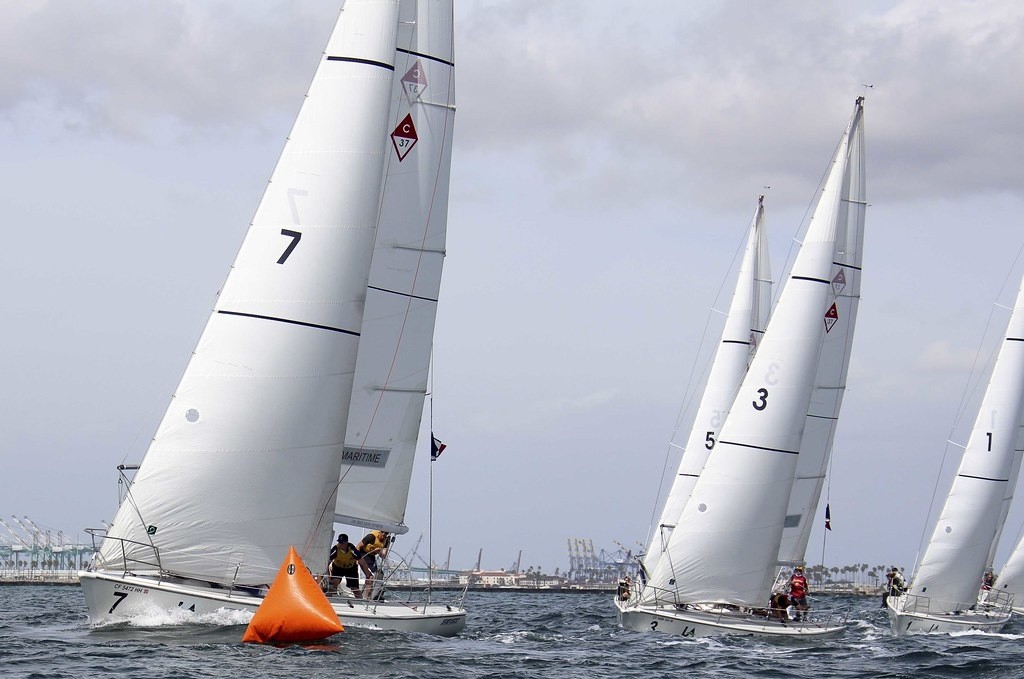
[611,90,869,646]
[75,0,472,640]
[885,249,1024,636]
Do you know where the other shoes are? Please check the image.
[374,596,385,600]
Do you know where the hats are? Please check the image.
[337,534,348,542]
[795,566,803,571]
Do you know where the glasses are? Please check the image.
[381,531,389,535]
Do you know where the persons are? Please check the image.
[618,577,631,601]
[981,571,995,612]
[879,567,909,610]
[330,533,372,598]
[356,529,390,599]
[772,594,794,622]
[787,566,809,621]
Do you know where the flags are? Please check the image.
[825,505,831,531]
[431,433,447,461]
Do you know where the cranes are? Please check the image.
[0,514,94,570]
[566,538,647,586]
[388,529,521,582]
